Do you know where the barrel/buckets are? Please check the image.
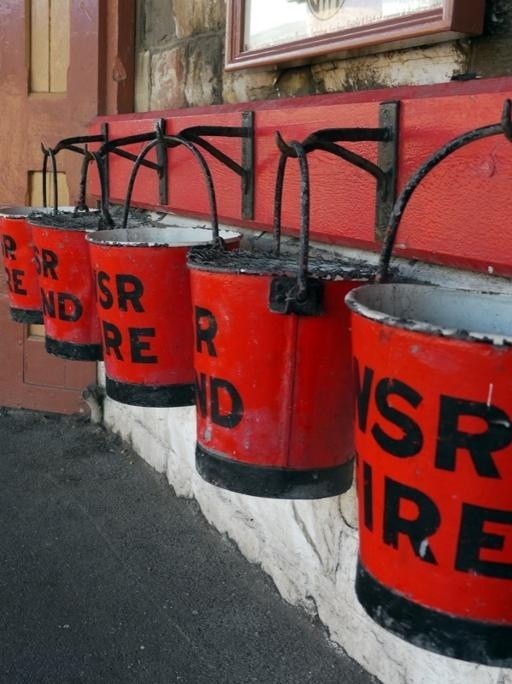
[343,121,512,669]
[184,140,396,500]
[83,137,245,407]
[25,150,149,361]
[0,148,97,323]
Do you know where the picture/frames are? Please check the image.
[225,0,484,73]
